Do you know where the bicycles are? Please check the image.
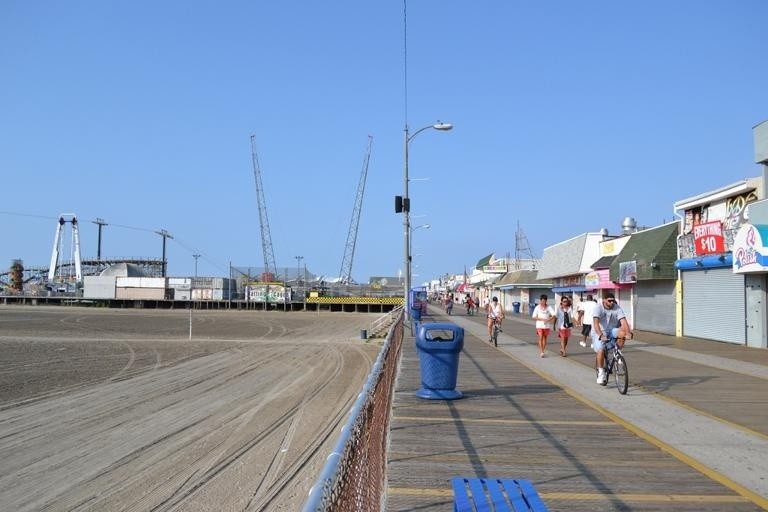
[487,313,506,348]
[595,332,634,394]
[469,307,475,316]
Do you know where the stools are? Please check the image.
[451,478,548,512]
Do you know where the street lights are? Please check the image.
[404,123,453,320]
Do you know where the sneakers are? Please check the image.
[541,349,565,357]
[597,372,607,385]
[580,341,593,348]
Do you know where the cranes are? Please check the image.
[251,135,278,284]
[339,136,373,281]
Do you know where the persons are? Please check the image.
[426,289,504,343]
[577,294,599,349]
[576,297,584,326]
[552,295,574,357]
[532,293,556,357]
[592,293,630,385]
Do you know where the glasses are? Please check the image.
[608,300,614,302]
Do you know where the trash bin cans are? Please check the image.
[411,303,422,321]
[512,301,520,314]
[416,322,464,401]
[528,303,538,317]
